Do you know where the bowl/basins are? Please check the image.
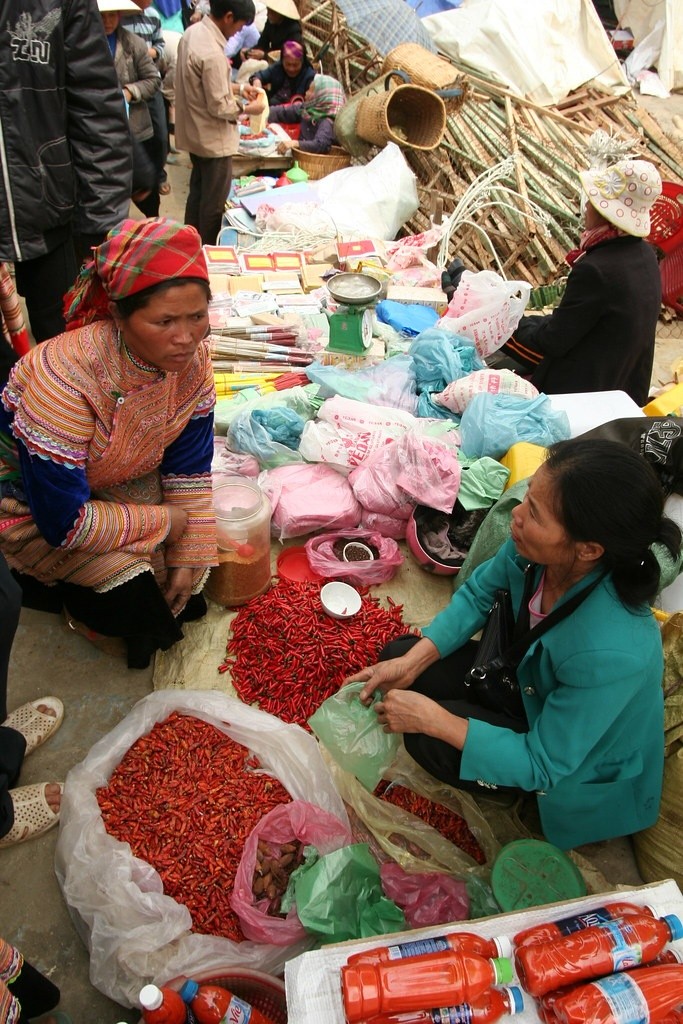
[320,582,362,619]
[327,272,382,304]
[342,541,374,562]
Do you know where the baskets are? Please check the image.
[138,968,286,1024]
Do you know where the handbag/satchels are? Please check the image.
[462,590,525,725]
[436,269,533,361]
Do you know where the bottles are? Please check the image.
[179,979,273,1024]
[202,475,272,605]
[138,984,196,1024]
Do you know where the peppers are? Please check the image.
[94,711,298,944]
[370,780,487,866]
[217,575,422,732]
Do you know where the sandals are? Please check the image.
[0,782,64,846]
[0,696,64,756]
[159,182,170,194]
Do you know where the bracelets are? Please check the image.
[240,84,244,97]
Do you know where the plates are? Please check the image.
[340,903,683,1024]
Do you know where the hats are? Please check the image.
[96,0,141,16]
[262,0,300,20]
[578,160,662,237]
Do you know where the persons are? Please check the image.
[484,160,664,409]
[338,439,683,852]
[0,696,66,851]
[1,216,219,670]
[1,0,350,386]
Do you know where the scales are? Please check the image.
[327,273,383,356]
